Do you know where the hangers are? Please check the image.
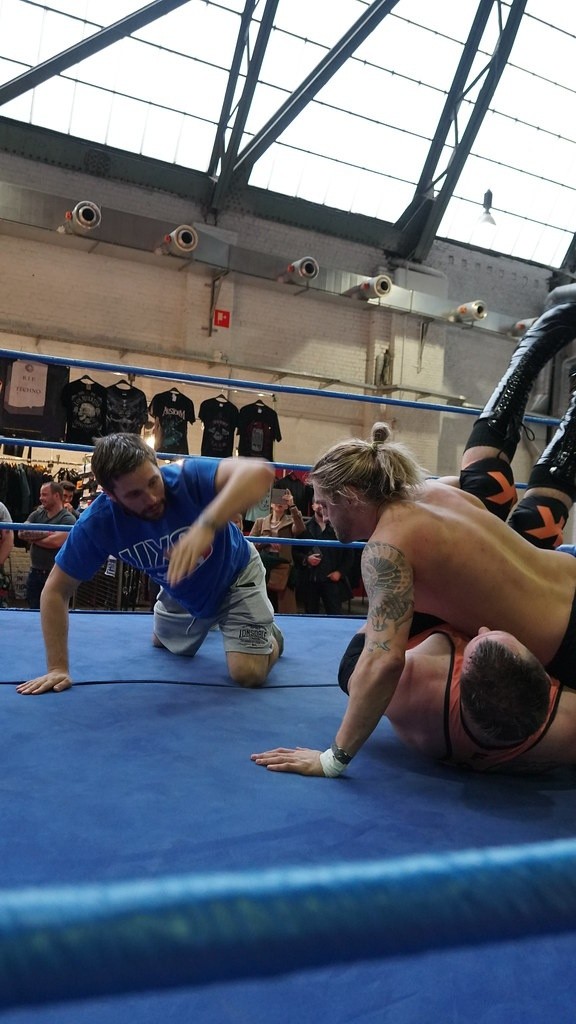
[254,387,266,404]
[115,372,131,387]
[215,385,228,401]
[167,379,181,394]
[80,369,97,383]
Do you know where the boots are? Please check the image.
[472,304,576,440]
[533,362,576,487]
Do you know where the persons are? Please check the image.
[231,513,243,530]
[338,283,575,770]
[292,495,346,614]
[37,480,80,518]
[15,432,283,695]
[249,488,306,613]
[18,482,77,609]
[0,502,16,608]
[250,423,576,776]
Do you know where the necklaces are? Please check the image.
[375,499,387,520]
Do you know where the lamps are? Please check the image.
[457,295,489,331]
[475,186,496,226]
[67,200,102,235]
[166,224,198,256]
[289,256,320,285]
[272,393,279,403]
[364,274,392,302]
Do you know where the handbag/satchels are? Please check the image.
[287,566,317,593]
[339,576,354,601]
[267,563,290,592]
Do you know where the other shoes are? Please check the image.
[271,622,284,656]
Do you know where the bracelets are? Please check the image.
[199,515,220,529]
[319,749,345,777]
[331,740,352,765]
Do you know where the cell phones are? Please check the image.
[272,489,288,504]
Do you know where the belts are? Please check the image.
[32,569,49,575]
